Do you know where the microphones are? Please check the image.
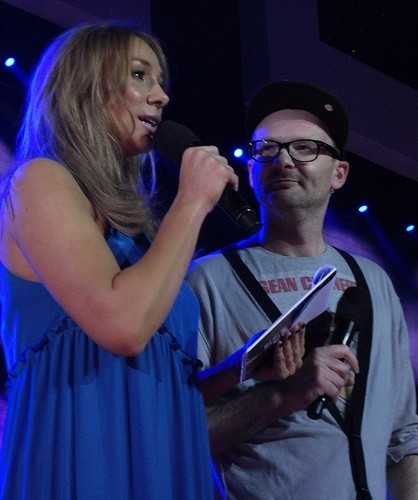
[154,121,260,233]
[307,286,371,420]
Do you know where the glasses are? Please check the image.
[247,138,342,162]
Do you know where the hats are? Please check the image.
[246,79,349,153]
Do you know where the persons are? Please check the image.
[181,107,418,500]
[0,13,309,500]
